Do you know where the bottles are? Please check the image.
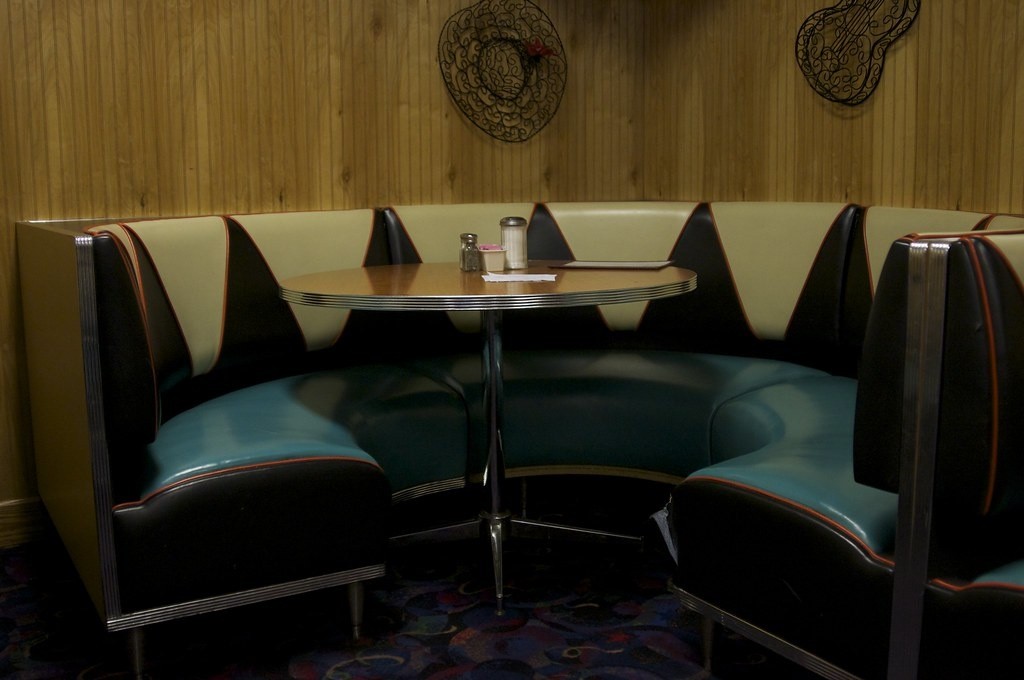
[499,216,527,269]
[459,233,481,272]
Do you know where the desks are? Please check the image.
[275,260,699,631]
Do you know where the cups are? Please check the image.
[478,248,508,274]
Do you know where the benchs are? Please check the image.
[15,200,1024,680]
